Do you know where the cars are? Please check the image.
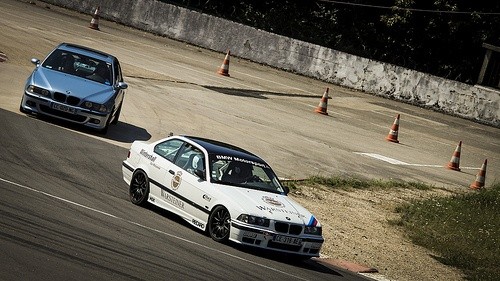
[121,135,325,261]
[20,43,128,135]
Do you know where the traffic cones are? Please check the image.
[316,87,329,116]
[470,159,488,190]
[446,141,463,171]
[217,50,230,77]
[385,114,401,143]
[88,6,100,30]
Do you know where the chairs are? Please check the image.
[182,154,205,169]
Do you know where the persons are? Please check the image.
[93,64,110,83]
[226,162,253,184]
[61,60,76,74]
